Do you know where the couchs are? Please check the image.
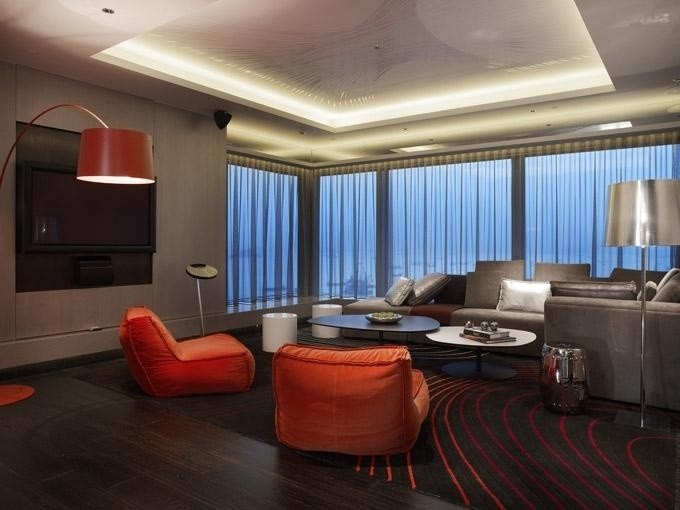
[340,256,680,412]
[271,340,431,459]
[116,305,255,397]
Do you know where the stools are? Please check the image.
[311,303,342,340]
[261,312,299,355]
[541,336,591,417]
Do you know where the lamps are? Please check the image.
[1,101,158,408]
[210,109,232,130]
[601,180,679,433]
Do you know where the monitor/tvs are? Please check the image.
[23,162,157,252]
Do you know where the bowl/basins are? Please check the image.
[365,311,404,324]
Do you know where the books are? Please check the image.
[458,332,516,345]
[463,326,509,340]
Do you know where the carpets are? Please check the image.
[56,318,679,509]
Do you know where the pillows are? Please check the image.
[464,268,507,310]
[383,274,415,307]
[495,277,551,315]
[406,265,450,306]
